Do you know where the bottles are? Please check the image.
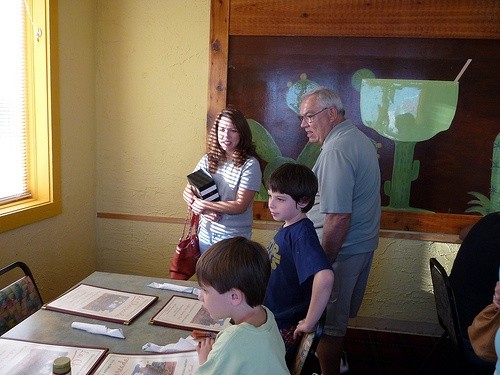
[52,357,72,375]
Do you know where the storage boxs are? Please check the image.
[187,169,221,202]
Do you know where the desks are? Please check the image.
[0,271,233,375]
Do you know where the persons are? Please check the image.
[297,89,381,375]
[449,212,500,375]
[183,108,268,255]
[263,164,335,375]
[196,236,290,375]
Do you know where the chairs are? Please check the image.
[414,258,470,375]
[0,261,43,336]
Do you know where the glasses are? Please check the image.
[298,108,329,122]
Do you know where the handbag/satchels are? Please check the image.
[169,198,199,281]
